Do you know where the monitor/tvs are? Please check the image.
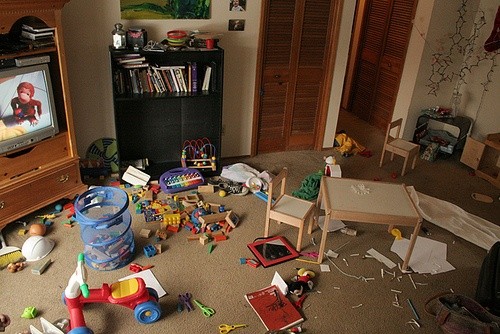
[0,64,60,153]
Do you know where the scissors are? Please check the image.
[193,299,215,317]
[178,292,194,312]
[219,323,246,334]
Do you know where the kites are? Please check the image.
[288,265,316,296]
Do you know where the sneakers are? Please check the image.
[218,183,249,196]
[208,176,238,187]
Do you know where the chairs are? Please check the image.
[264,167,316,252]
[379,117,421,176]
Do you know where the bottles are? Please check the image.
[112,22,126,50]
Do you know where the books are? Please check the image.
[115,53,217,94]
[21,24,55,40]
[244,285,304,332]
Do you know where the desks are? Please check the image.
[316,175,424,269]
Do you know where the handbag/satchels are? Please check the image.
[424,293,500,334]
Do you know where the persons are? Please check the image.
[234,20,244,30]
[232,0,244,11]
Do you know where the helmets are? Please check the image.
[22,236,55,262]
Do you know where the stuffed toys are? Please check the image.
[19,305,38,320]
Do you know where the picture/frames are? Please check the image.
[247,233,300,268]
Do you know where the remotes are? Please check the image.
[32,258,52,275]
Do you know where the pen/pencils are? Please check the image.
[406,297,419,320]
[293,267,300,270]
[274,289,284,306]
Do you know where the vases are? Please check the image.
[446,90,463,118]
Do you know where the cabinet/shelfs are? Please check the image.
[459,132,499,188]
[109,45,224,181]
[0,0,89,228]
[409,105,472,157]
